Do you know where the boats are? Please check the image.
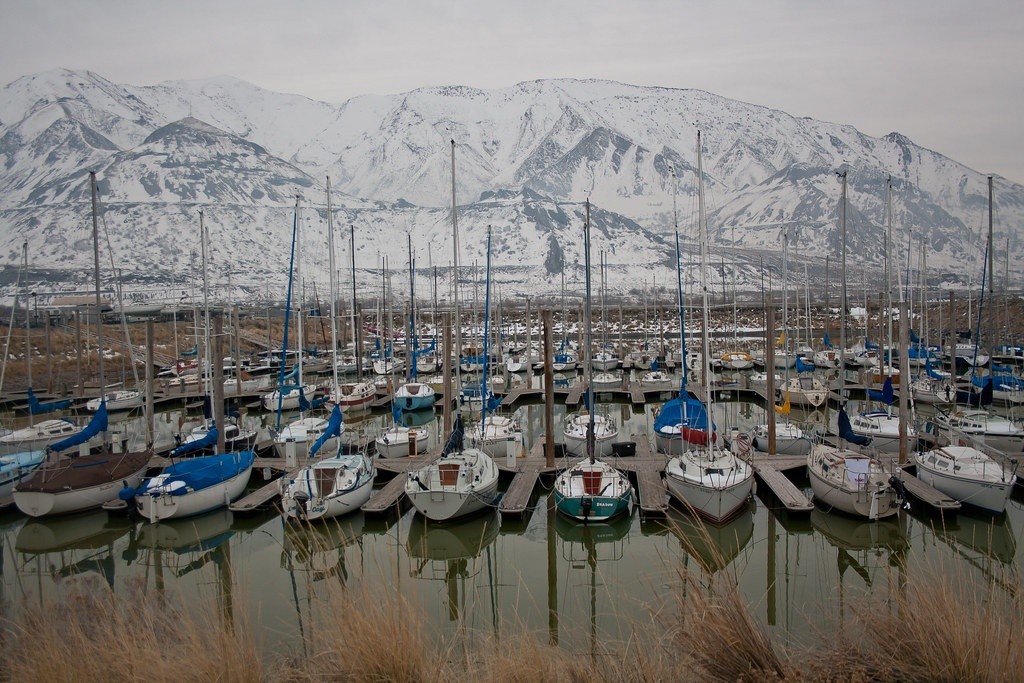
[900,495,1016,565]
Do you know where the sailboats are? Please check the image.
[0,129,1024,530]
[279,507,366,662]
[665,497,758,625]
[551,505,633,670]
[408,502,500,638]
[16,508,137,613]
[121,507,236,610]
[806,492,908,646]
